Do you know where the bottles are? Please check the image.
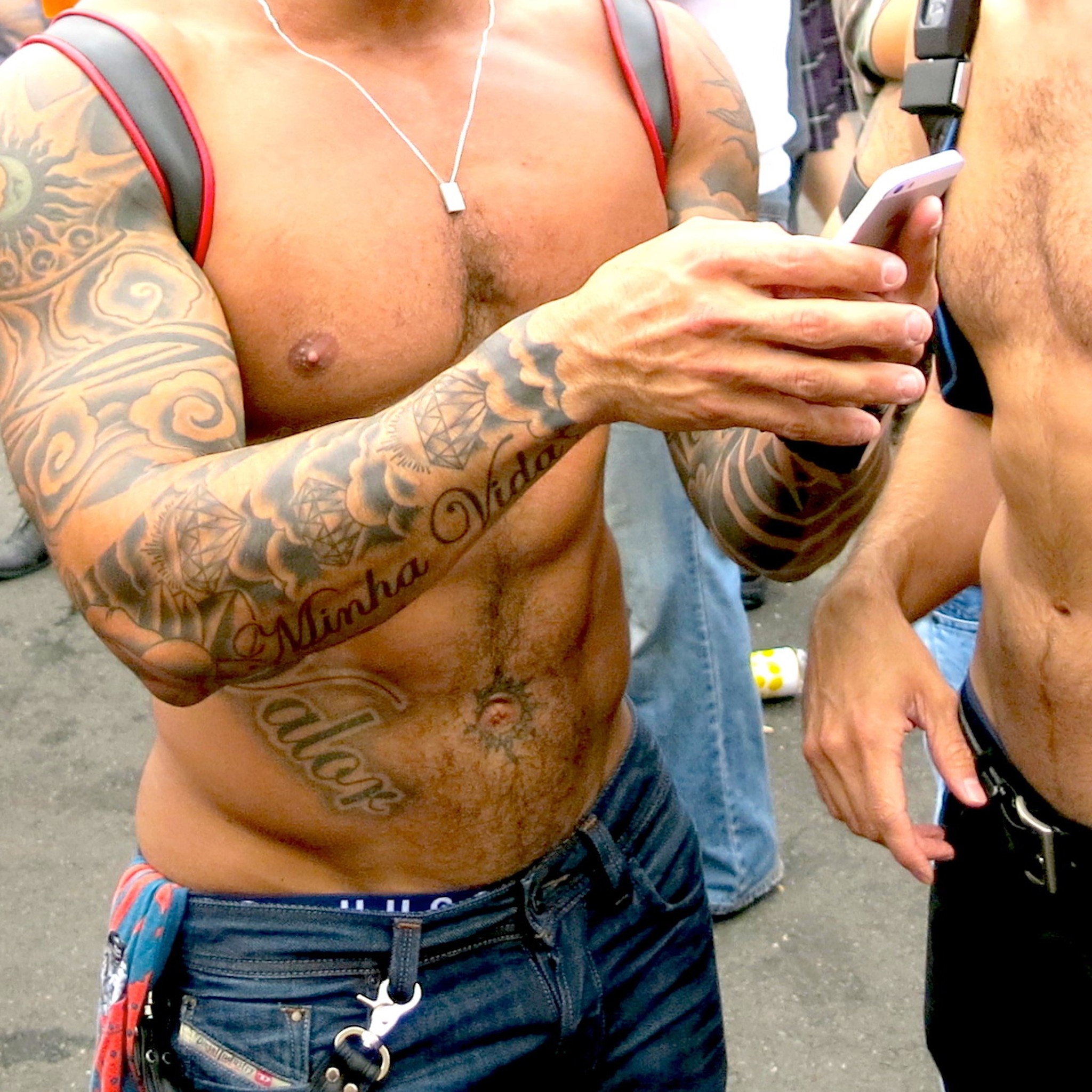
[750,646,808,699]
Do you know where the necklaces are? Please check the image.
[258,0,496,213]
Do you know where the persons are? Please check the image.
[797,0,1091,1092]
[0,0,85,579]
[0,0,947,1092]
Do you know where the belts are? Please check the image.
[957,699,1092,901]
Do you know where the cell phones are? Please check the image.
[794,148,963,302]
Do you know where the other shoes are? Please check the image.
[0,510,52,580]
